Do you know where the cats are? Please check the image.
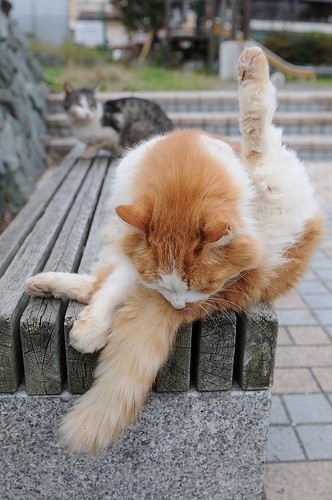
[26,46,324,452]
[61,78,174,159]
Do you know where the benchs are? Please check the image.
[0,140,278,500]
